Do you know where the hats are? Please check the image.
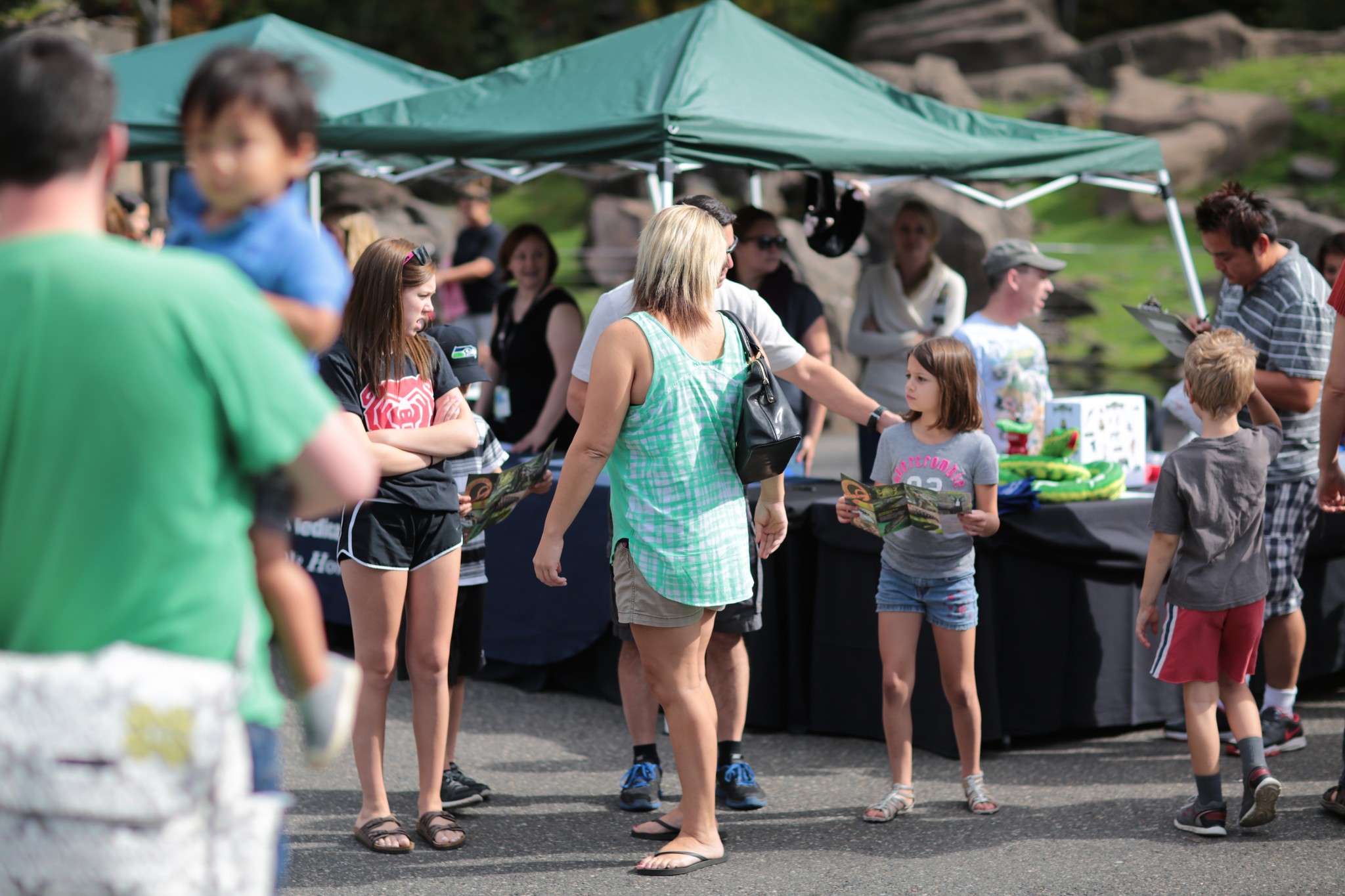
[981,237,1067,275]
[425,324,494,385]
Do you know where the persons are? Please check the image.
[566,193,906,811]
[948,238,1067,458]
[473,221,586,455]
[426,323,553,806]
[835,337,1001,823]
[0,29,379,893]
[531,203,788,878]
[158,41,366,766]
[1134,325,1285,835]
[723,203,833,479]
[1318,253,1345,816]
[433,179,510,398]
[111,189,166,250]
[846,197,968,480]
[1317,231,1345,290]
[1177,178,1338,759]
[316,235,479,855]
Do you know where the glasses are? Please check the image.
[744,235,787,249]
[404,244,433,266]
[726,234,738,254]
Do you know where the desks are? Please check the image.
[481,445,1345,760]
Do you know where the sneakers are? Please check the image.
[301,652,363,766]
[1238,765,1282,827]
[1173,794,1228,835]
[1225,707,1307,757]
[1164,706,1234,743]
[441,761,490,808]
[620,761,663,811]
[715,753,766,809]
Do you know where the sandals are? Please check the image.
[414,811,466,851]
[863,783,915,821]
[961,770,999,813]
[355,815,415,854]
[1319,785,1345,816]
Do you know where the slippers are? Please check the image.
[632,818,728,841]
[637,850,729,875]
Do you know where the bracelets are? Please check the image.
[867,405,888,432]
[429,455,434,467]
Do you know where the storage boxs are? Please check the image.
[1044,391,1151,490]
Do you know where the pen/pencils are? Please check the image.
[1199,314,1212,325]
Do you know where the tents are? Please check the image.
[89,8,461,169]
[317,0,1207,328]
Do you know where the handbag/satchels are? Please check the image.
[715,310,802,485]
[2,651,291,896]
